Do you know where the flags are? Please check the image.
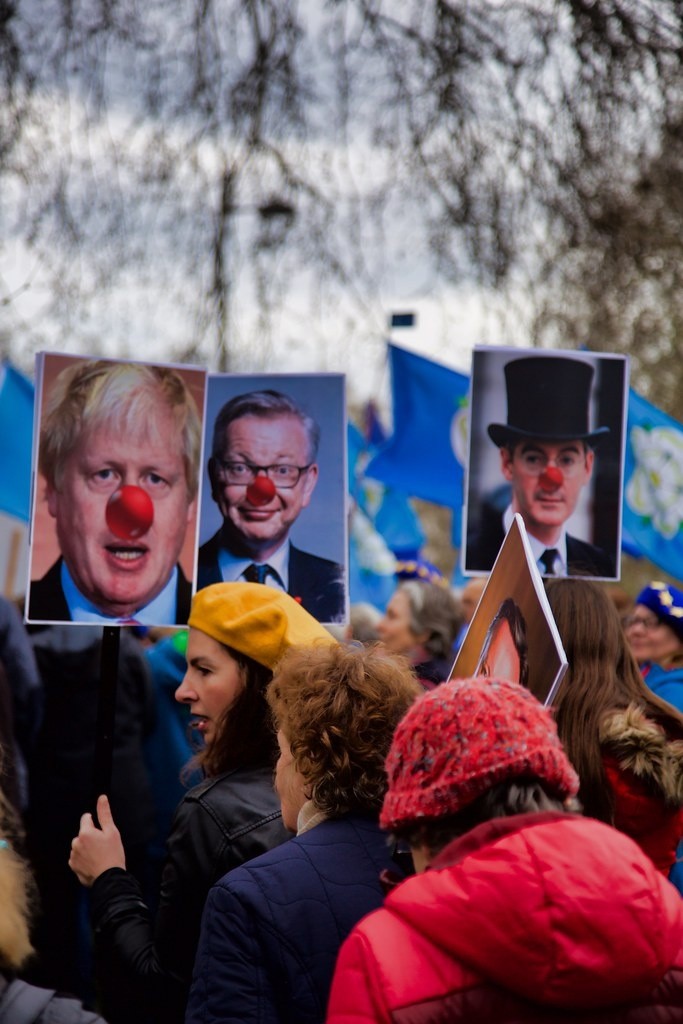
[335,342,682,623]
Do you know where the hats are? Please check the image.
[635,580,683,631]
[488,355,613,450]
[380,674,580,829]
[189,582,337,673]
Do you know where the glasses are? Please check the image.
[509,450,588,474]
[211,453,317,489]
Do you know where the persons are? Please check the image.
[470,354,617,580]
[471,597,528,688]
[30,358,198,625]
[197,392,344,624]
[0,581,683,1024]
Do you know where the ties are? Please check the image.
[540,548,560,576]
[242,564,272,584]
